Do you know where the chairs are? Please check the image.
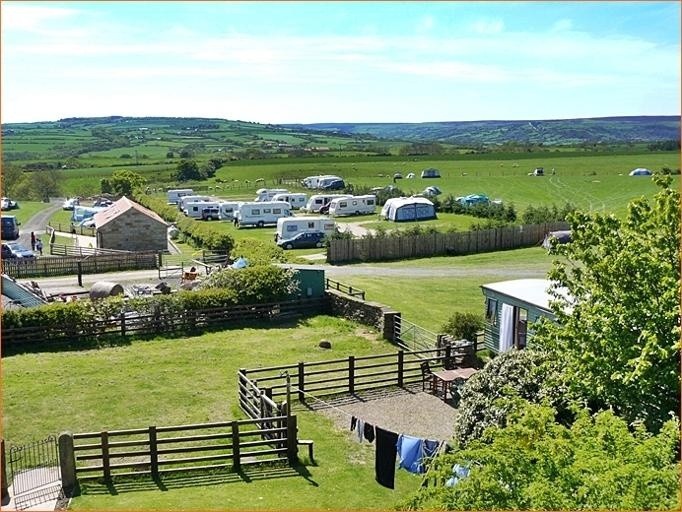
[420,357,457,395]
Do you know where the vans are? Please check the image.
[277,216,334,240]
[167,189,242,222]
[329,195,376,216]
[1,216,21,239]
[255,189,308,209]
[307,195,353,215]
[237,201,290,227]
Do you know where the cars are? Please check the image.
[72,205,84,221]
[1,198,17,210]
[63,198,79,210]
[2,242,34,264]
[281,231,329,250]
[95,198,112,206]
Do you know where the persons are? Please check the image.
[70,222,77,233]
[31,232,43,253]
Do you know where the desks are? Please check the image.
[431,367,478,402]
